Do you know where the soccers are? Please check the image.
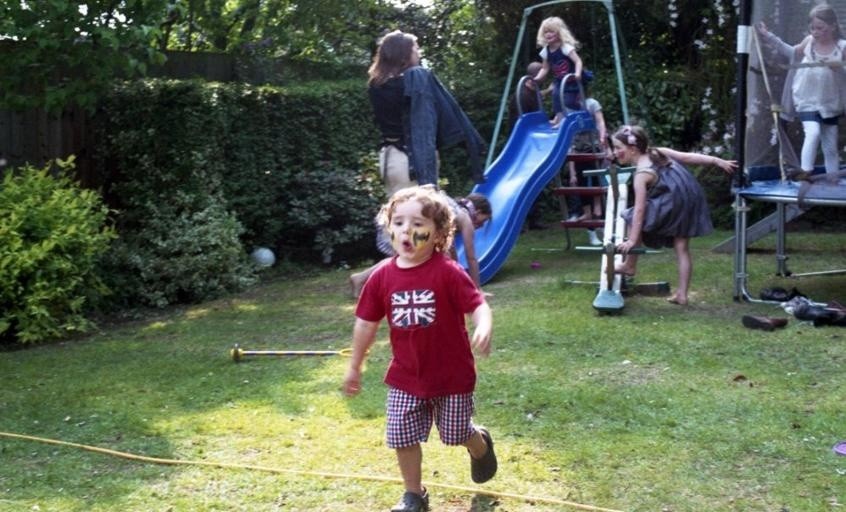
[248,248,276,269]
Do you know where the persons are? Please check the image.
[366,29,422,260]
[447,191,492,296]
[562,131,603,221]
[524,14,595,117]
[756,3,846,185]
[548,109,564,131]
[558,83,609,224]
[341,182,498,512]
[603,125,741,306]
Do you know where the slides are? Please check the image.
[455,74,595,285]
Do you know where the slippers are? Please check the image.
[390,485,431,512]
[468,425,498,484]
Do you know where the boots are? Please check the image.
[743,314,788,333]
[794,304,838,327]
